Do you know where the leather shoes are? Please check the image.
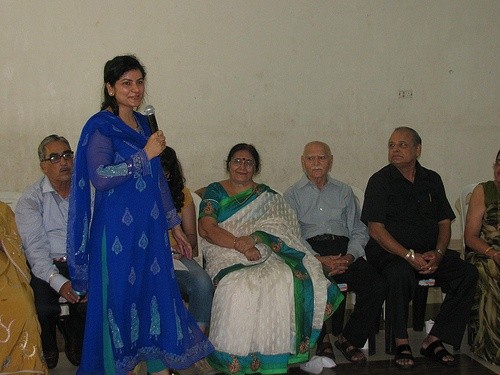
[42,340,59,369]
[58,315,80,365]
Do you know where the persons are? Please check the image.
[360,126,479,369]
[198,143,344,375]
[1,199,51,375]
[158,146,214,374]
[15,134,86,370]
[464,149,500,366]
[282,141,389,362]
[65,55,215,375]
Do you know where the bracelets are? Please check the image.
[491,251,500,259]
[403,248,415,260]
[434,249,445,256]
[250,234,259,244]
[233,236,237,249]
[484,246,494,256]
[49,273,60,284]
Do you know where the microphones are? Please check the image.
[144,104,158,134]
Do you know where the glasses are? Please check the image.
[230,159,253,168]
[44,151,74,164]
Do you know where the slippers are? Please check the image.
[318,342,334,359]
[334,340,366,364]
[395,344,413,368]
[421,340,454,363]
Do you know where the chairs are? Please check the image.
[56,261,446,355]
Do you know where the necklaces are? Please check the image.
[228,179,256,205]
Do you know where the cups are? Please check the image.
[425,321,434,334]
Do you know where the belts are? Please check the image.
[312,233,342,240]
[54,257,66,262]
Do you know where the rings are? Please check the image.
[67,292,70,295]
